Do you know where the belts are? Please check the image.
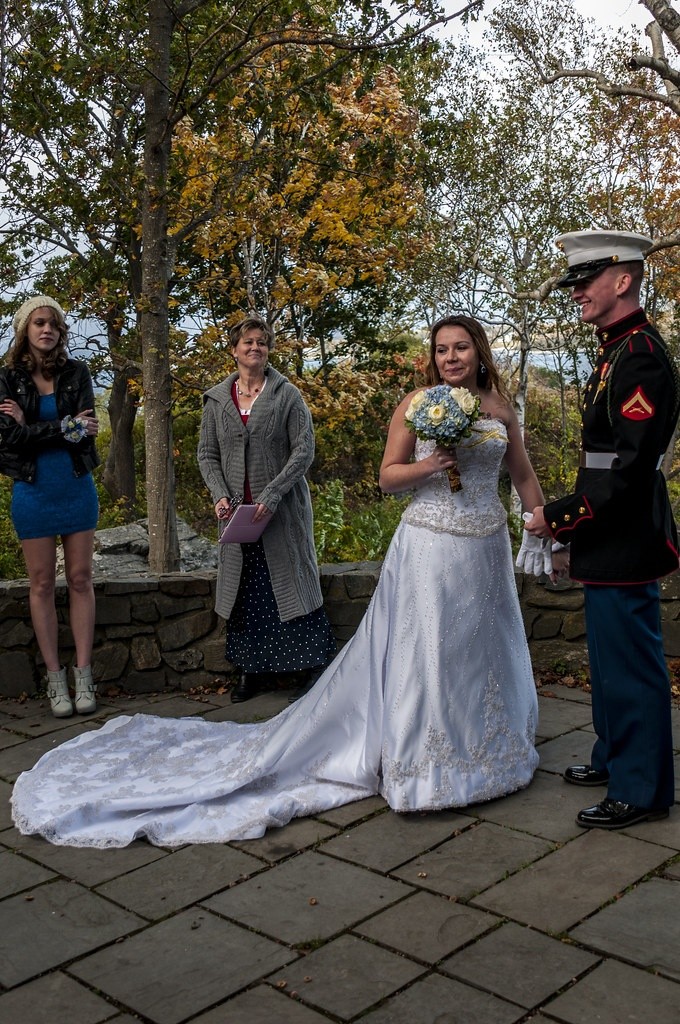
[577,449,665,470]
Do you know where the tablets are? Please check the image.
[218,505,274,543]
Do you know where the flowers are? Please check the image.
[402,383,481,494]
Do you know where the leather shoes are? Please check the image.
[288,666,322,703]
[575,794,669,828]
[563,763,612,785]
[230,670,261,703]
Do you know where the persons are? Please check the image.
[197,318,337,701]
[10,314,569,847]
[0,297,100,716]
[524,231,680,828]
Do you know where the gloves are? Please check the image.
[514,511,570,576]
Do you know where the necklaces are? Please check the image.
[237,376,265,397]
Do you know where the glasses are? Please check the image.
[218,492,244,518]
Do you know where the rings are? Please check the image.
[450,450,453,455]
[13,409,16,413]
[262,512,268,515]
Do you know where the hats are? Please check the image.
[12,294,64,333]
[556,230,653,288]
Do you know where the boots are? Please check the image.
[73,664,98,713]
[45,666,72,718]
